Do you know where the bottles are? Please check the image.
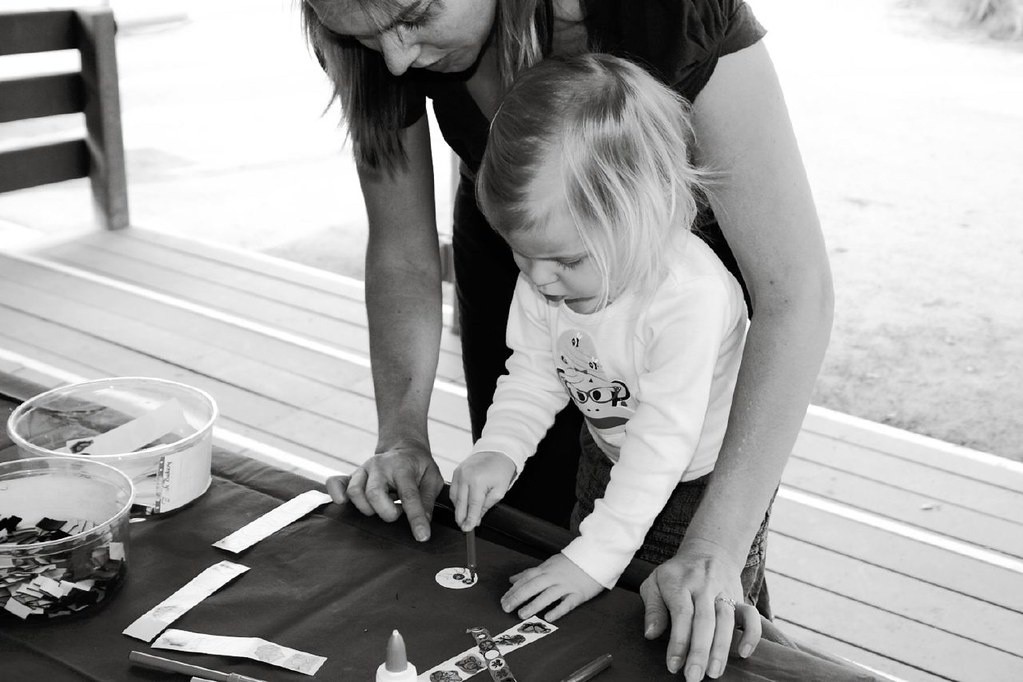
[375,629,418,682]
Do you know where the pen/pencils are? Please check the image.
[465,528,478,584]
[127,650,265,682]
[560,653,613,682]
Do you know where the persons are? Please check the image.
[451,55,768,622]
[302,0,838,682]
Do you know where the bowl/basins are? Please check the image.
[6,377,218,516]
[0,457,135,627]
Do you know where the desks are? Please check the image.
[0,372,885,682]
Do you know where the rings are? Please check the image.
[714,596,737,612]
